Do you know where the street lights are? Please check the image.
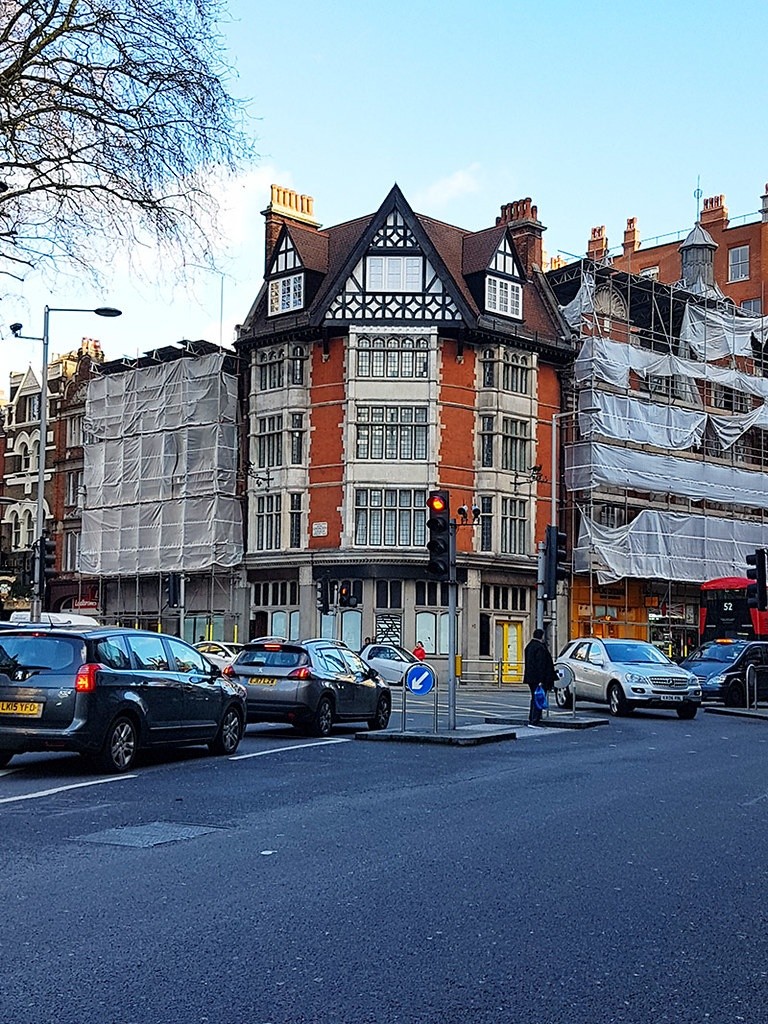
[9,305,123,621]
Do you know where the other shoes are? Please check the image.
[528,720,545,729]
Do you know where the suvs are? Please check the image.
[0,611,248,773]
[223,636,391,739]
[681,637,768,708]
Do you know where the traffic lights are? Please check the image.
[40,539,59,577]
[746,549,768,612]
[425,489,451,582]
[338,584,352,607]
[544,525,569,600]
[316,573,331,614]
[165,573,179,608]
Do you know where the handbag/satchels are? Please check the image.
[534,686,548,709]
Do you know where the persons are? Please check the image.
[523,629,557,721]
[363,637,371,648]
[412,641,425,661]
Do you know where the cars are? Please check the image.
[358,643,441,688]
[555,636,703,720]
